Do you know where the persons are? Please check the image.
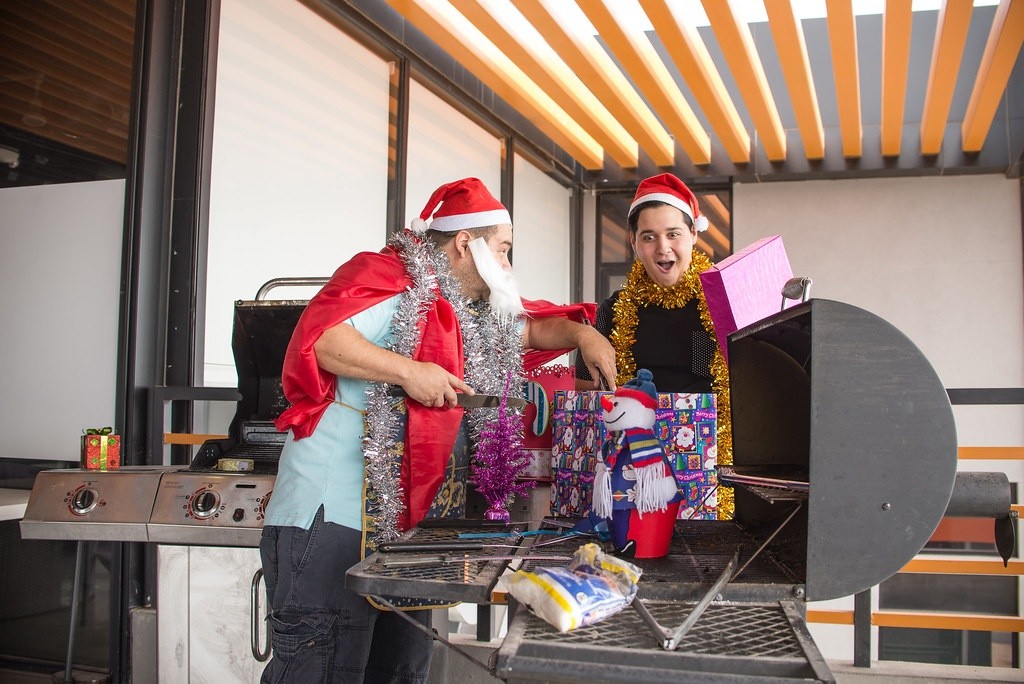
[260,178,617,684]
[575,172,719,394]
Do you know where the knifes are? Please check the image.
[376,553,571,568]
[420,518,532,526]
[387,384,533,410]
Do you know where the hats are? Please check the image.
[411,177,512,236]
[627,172,709,232]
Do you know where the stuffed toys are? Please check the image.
[590,370,684,558]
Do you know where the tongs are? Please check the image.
[626,541,743,650]
